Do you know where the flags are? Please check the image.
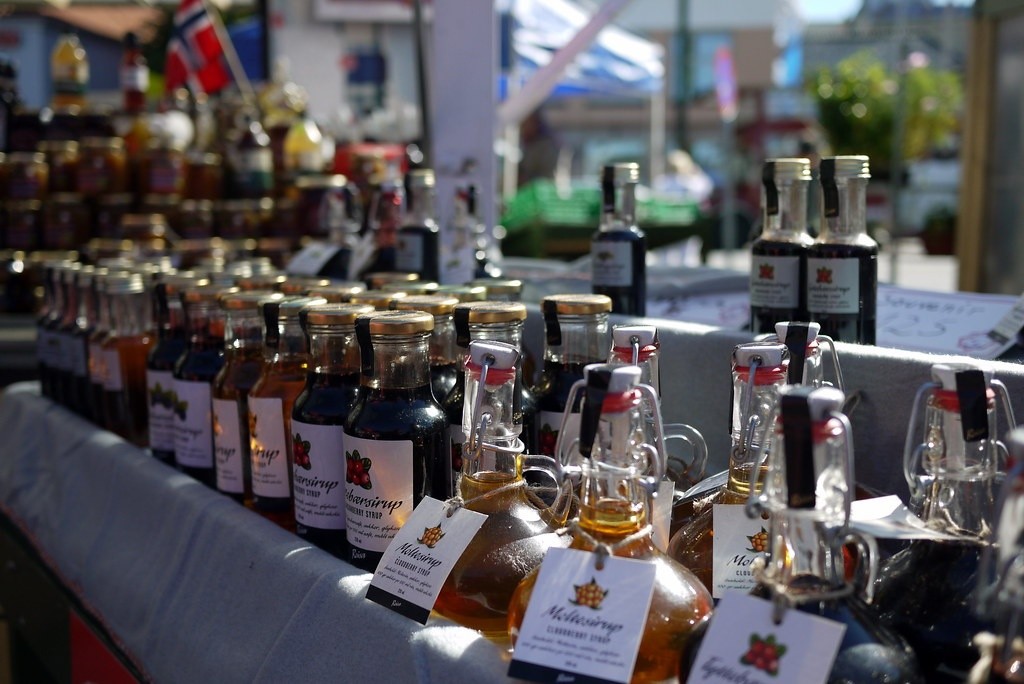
[165,0,230,96]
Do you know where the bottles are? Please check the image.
[0,26,1023,684]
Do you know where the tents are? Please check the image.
[493,0,664,192]
